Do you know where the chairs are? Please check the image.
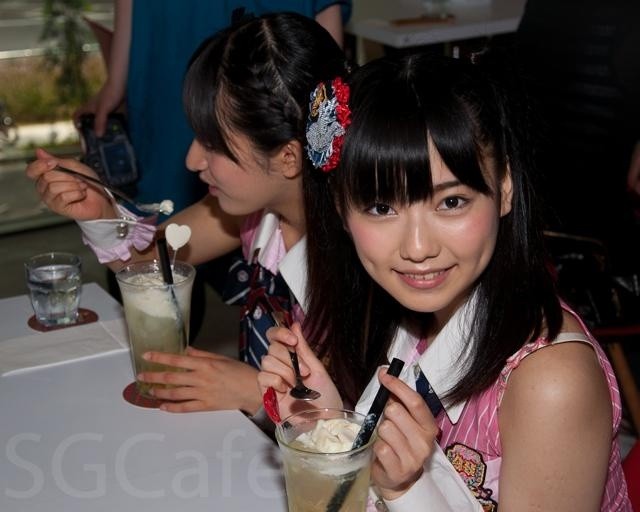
[587,322,640,512]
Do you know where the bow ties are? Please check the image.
[222,260,291,370]
[416,369,442,417]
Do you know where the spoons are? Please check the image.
[21,155,176,215]
[271,310,323,403]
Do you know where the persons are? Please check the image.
[513,0,640,441]
[258,52,633,512]
[24,12,359,447]
[70,0,353,344]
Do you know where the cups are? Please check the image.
[22,250,84,328]
[114,258,198,400]
[274,406,380,511]
